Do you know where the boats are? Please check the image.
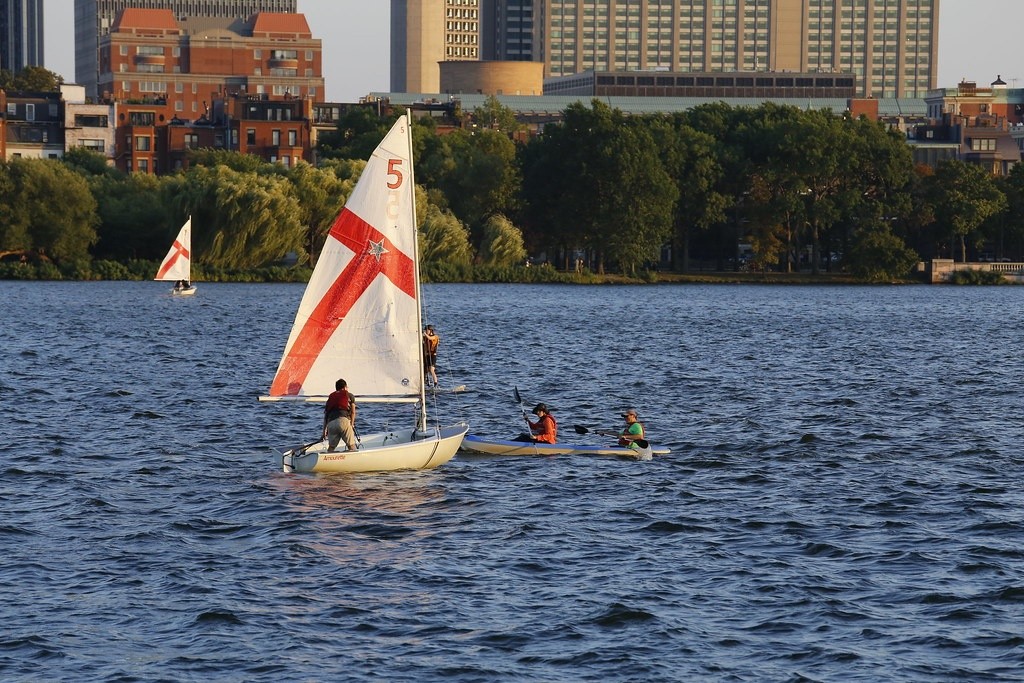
[426,380,468,396]
[460,434,673,457]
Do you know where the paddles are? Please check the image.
[574,424,649,449]
[514,385,539,454]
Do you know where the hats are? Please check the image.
[621,409,636,417]
[532,403,546,414]
[423,325,434,330]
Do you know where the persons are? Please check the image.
[511,403,556,444]
[173,280,190,291]
[599,410,644,449]
[422,325,439,388]
[321,379,357,452]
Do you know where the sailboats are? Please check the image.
[256,108,471,473]
[153,214,197,296]
